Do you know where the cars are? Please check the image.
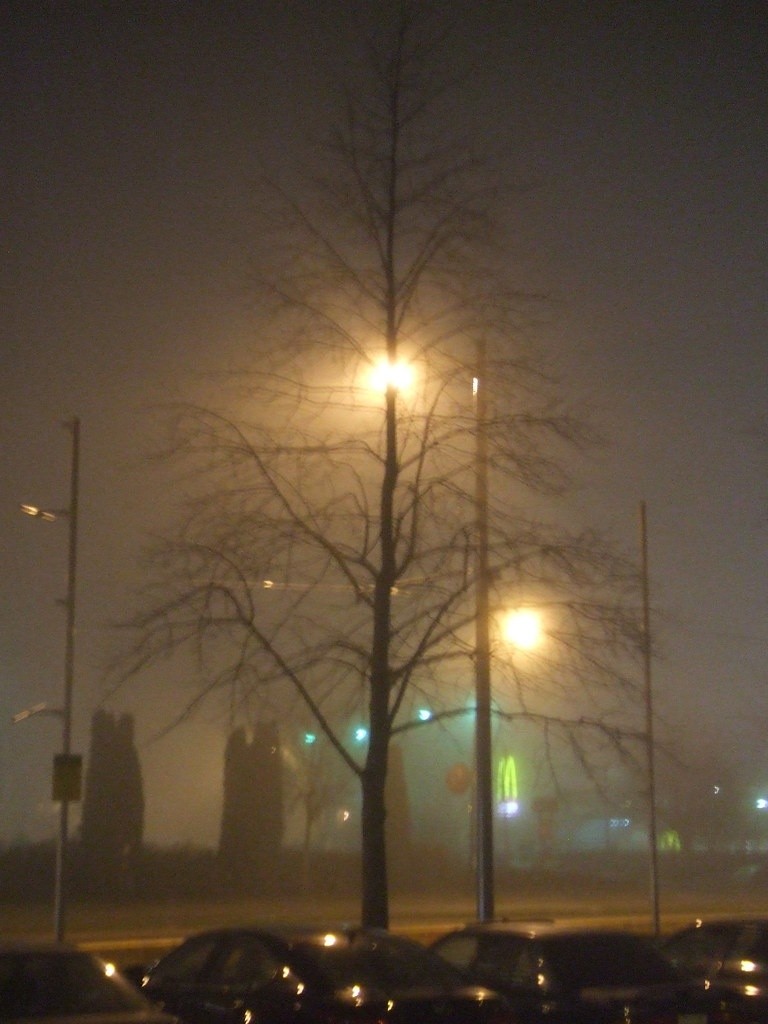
[421,912,731,1024]
[0,943,184,1024]
[123,917,518,1024]
[653,912,767,1024]
